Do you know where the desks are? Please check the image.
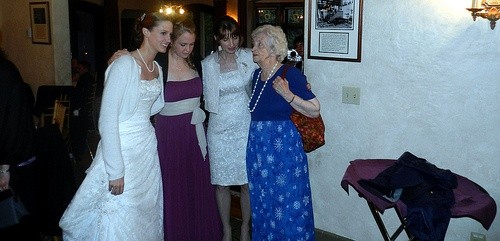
[343,158,496,241]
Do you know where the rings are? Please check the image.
[2,186,3,190]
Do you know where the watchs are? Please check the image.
[0,169,10,174]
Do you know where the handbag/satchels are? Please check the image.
[280,64,326,153]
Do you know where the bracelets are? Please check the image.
[288,94,295,104]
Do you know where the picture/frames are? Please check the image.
[307,0,364,62]
[29,2,52,44]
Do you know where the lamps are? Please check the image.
[466,0,500,30]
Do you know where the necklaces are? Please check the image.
[220,52,237,72]
[247,58,279,113]
[136,48,155,72]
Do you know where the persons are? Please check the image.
[72,56,96,90]
[245,25,324,241]
[197,15,311,241]
[59,14,173,241]
[109,19,226,241]
[0,45,30,241]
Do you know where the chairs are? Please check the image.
[36,78,105,170]
